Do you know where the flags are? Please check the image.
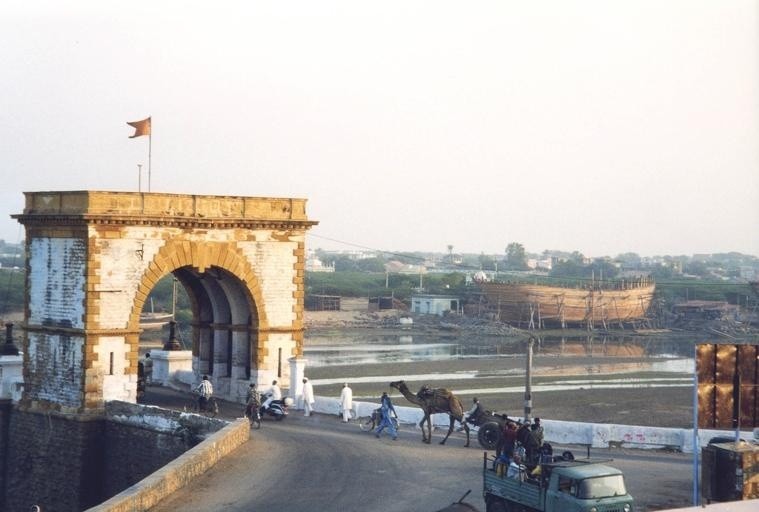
[126,117,151,137]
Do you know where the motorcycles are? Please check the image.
[260,393,293,421]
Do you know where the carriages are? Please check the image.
[390,381,524,450]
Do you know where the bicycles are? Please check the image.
[182,391,219,418]
[241,402,261,429]
[359,409,399,434]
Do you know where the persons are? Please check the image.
[193,375,213,410]
[374,391,398,440]
[340,383,353,424]
[455,397,483,432]
[245,383,260,417]
[262,379,281,409]
[301,376,314,417]
[493,417,544,483]
[143,353,153,387]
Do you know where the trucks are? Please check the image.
[484,444,634,512]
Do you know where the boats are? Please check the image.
[481,274,656,325]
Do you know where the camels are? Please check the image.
[389,381,470,447]
